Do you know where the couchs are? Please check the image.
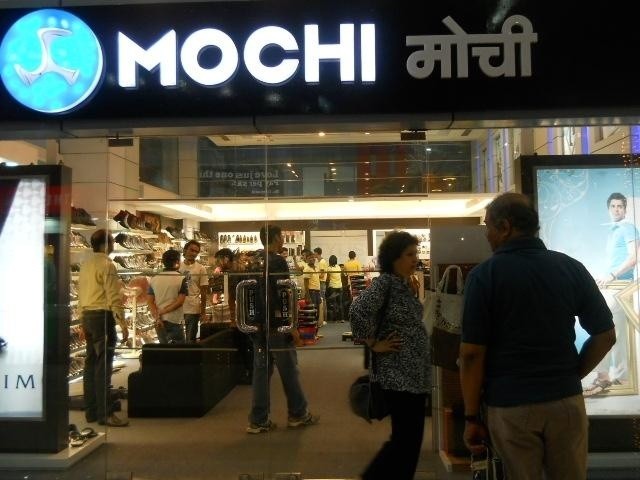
[200,323,273,384]
[128,320,236,418]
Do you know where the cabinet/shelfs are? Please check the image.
[67,206,126,383]
[109,209,219,358]
[218,231,305,256]
[350,257,380,300]
[404,229,430,260]
[211,249,318,345]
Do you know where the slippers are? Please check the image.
[81,427,97,438]
[71,436,87,447]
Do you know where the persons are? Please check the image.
[458,193,616,475]
[338,263,344,271]
[297,250,313,272]
[278,247,288,262]
[177,240,209,340]
[79,228,129,427]
[345,251,365,296]
[303,252,323,340]
[327,255,344,322]
[353,231,431,480]
[246,225,320,433]
[313,248,327,326]
[582,194,639,389]
[147,249,189,344]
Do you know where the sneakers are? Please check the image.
[286,411,319,427]
[246,419,275,433]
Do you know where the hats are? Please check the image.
[350,275,389,422]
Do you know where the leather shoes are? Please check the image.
[224,235,228,243]
[98,414,129,427]
[114,256,125,268]
[220,235,224,243]
[121,306,159,347]
[239,235,242,243]
[247,236,249,243]
[291,235,295,242]
[173,229,188,239]
[125,210,145,230]
[123,254,155,269]
[236,235,239,243]
[68,204,96,377]
[124,235,153,249]
[166,226,175,237]
[114,234,127,248]
[228,235,231,243]
[113,210,128,228]
[254,235,257,243]
[85,415,97,423]
[250,235,253,243]
[286,235,289,242]
[243,235,246,243]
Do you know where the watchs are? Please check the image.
[370,338,379,348]
[465,415,482,422]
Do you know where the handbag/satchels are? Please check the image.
[470,438,504,476]
[423,266,466,370]
